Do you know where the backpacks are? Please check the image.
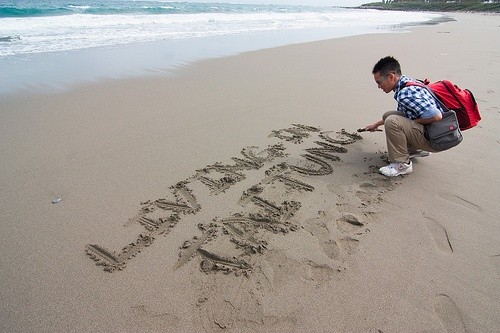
[399,79,481,131]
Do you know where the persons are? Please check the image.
[362,56,445,178]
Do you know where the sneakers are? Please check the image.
[409,150,430,158]
[379,160,413,177]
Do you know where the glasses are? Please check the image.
[376,72,395,84]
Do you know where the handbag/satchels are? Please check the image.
[422,110,464,151]
